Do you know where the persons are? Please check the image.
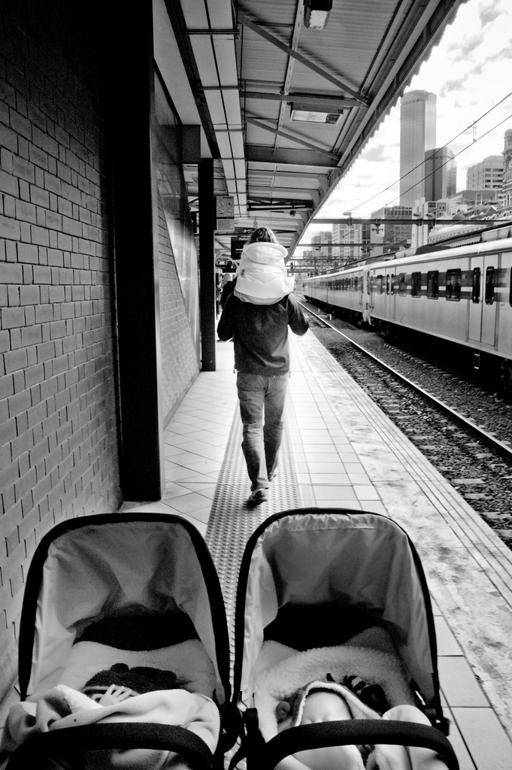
[99,684,134,706]
[297,689,351,729]
[217,285,310,510]
[234,227,291,306]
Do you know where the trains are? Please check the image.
[302,219,512,393]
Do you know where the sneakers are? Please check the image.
[246,488,268,505]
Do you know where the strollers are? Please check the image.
[0,510,236,770]
[229,508,459,769]
